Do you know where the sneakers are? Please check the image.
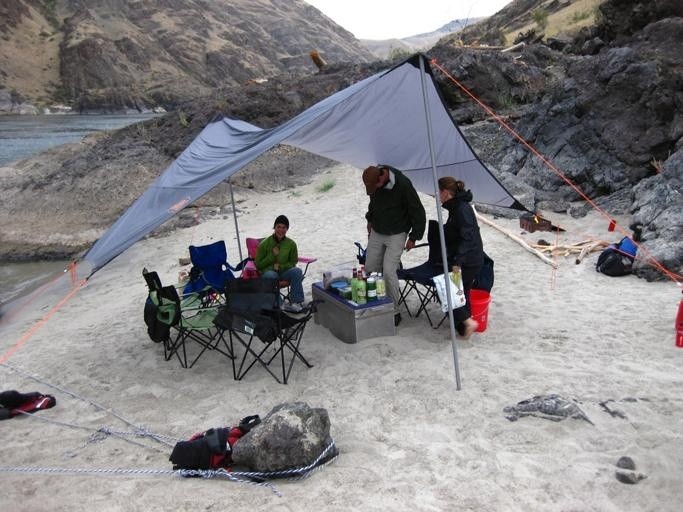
[395,313,402,326]
[284,301,302,312]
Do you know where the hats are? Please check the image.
[362,165,380,196]
[274,215,289,229]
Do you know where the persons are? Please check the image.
[360,164,426,330]
[253,213,305,313]
[434,175,485,340]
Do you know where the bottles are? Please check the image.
[351,267,386,305]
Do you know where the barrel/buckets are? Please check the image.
[468,289,491,332]
